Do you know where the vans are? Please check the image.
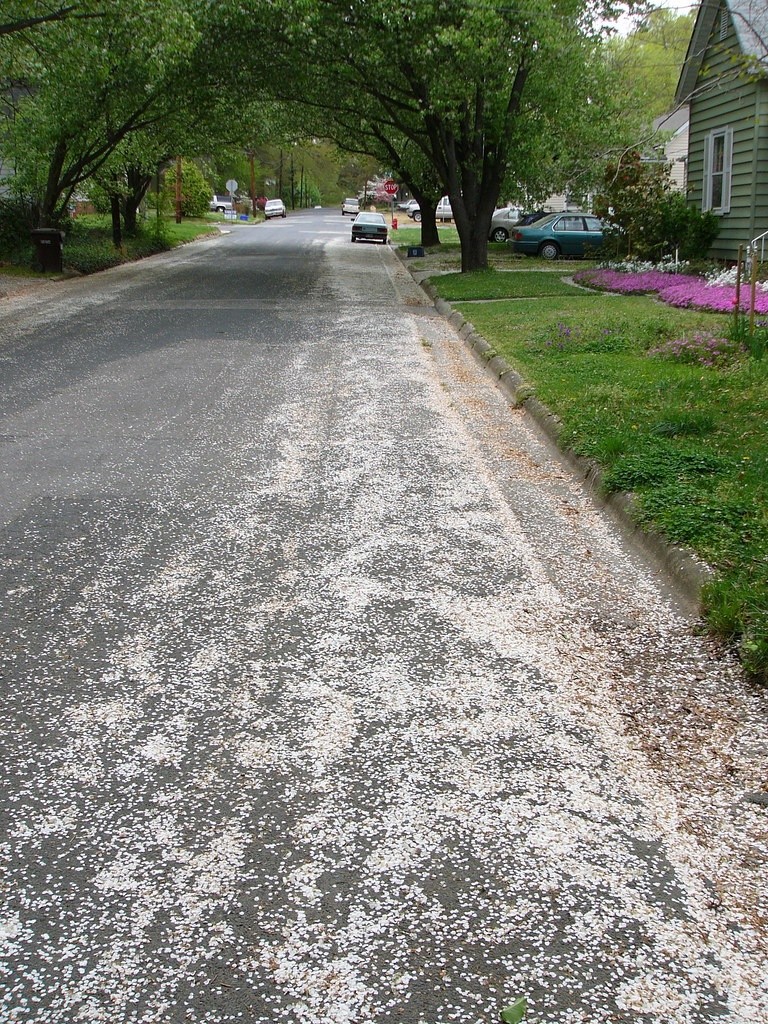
[407,195,455,222]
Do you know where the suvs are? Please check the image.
[264,198,287,220]
[341,197,360,216]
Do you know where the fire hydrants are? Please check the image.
[391,218,398,230]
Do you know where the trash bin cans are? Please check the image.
[30,228,66,273]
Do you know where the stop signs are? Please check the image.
[383,180,399,195]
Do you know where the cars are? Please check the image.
[510,212,573,232]
[487,206,552,243]
[350,212,388,244]
[396,199,410,210]
[505,211,629,262]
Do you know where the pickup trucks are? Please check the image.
[207,194,232,213]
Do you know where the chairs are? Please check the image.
[567,221,582,230]
